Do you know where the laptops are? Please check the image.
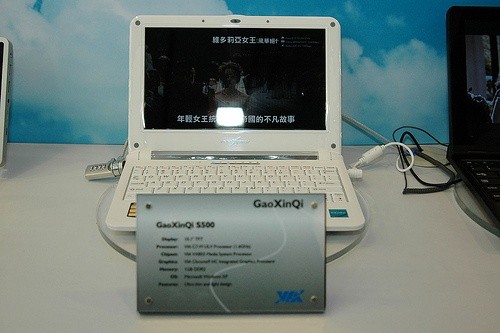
[446,6,500,223]
[105,15,366,231]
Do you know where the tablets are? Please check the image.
[0,37,13,165]
